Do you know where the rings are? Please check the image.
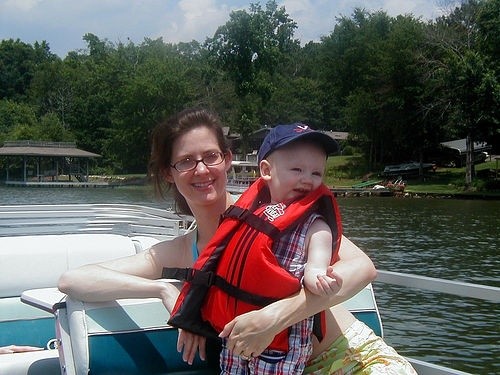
[240,352,249,360]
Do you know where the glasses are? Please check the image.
[168,152,226,173]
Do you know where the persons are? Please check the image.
[55,107,419,375]
[167,123,343,375]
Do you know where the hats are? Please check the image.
[257,121,339,168]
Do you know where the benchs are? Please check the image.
[1,234,383,375]
